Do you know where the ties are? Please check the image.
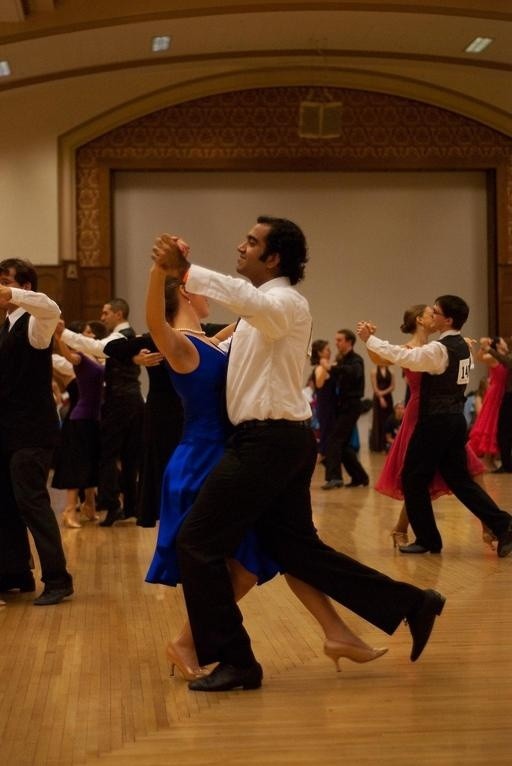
[1,315,11,348]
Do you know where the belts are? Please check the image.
[234,419,312,432]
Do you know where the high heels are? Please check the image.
[391,529,410,549]
[60,509,83,529]
[322,638,389,673]
[78,502,101,523]
[482,529,500,551]
[165,641,210,680]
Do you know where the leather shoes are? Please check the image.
[135,513,157,528]
[98,508,124,526]
[498,512,512,558]
[479,460,508,474]
[33,572,74,605]
[345,475,369,487]
[405,586,447,664]
[398,536,442,555]
[187,661,263,691]
[0,571,36,594]
[320,479,344,489]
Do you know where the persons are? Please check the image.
[150,216,446,694]
[50,295,512,559]
[147,234,389,683]
[0,258,77,606]
